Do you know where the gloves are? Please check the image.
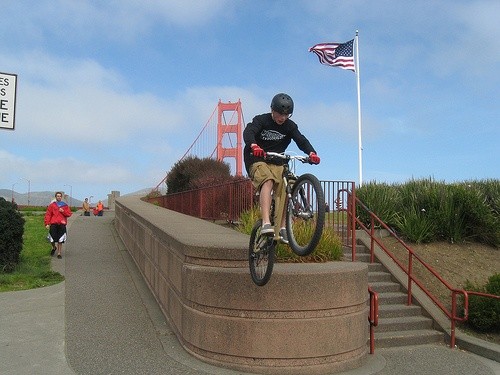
[309,152,320,164]
[251,145,266,160]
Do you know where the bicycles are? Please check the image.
[248,151,325,286]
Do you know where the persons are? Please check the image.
[243,93,320,243]
[83,198,90,216]
[93,200,104,216]
[44,192,72,259]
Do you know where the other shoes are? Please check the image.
[51,247,57,255]
[58,255,62,259]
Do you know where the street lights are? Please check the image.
[89,196,93,203]
[64,185,72,205]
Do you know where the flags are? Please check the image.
[307,38,355,72]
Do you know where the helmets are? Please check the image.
[271,93,293,114]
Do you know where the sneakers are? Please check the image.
[260,224,274,235]
[279,229,288,244]
